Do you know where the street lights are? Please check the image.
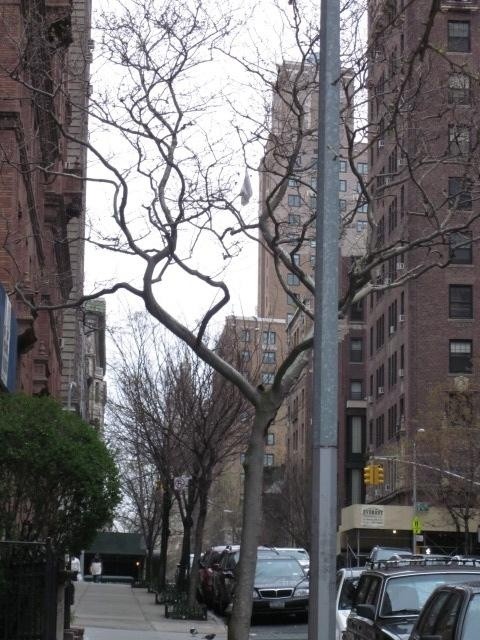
[412,428,426,553]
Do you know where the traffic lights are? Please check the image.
[155,480,165,498]
[363,463,386,486]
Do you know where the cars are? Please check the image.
[334,547,479,639]
[175,545,311,624]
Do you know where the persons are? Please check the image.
[71,553,81,584]
[88,553,103,584]
